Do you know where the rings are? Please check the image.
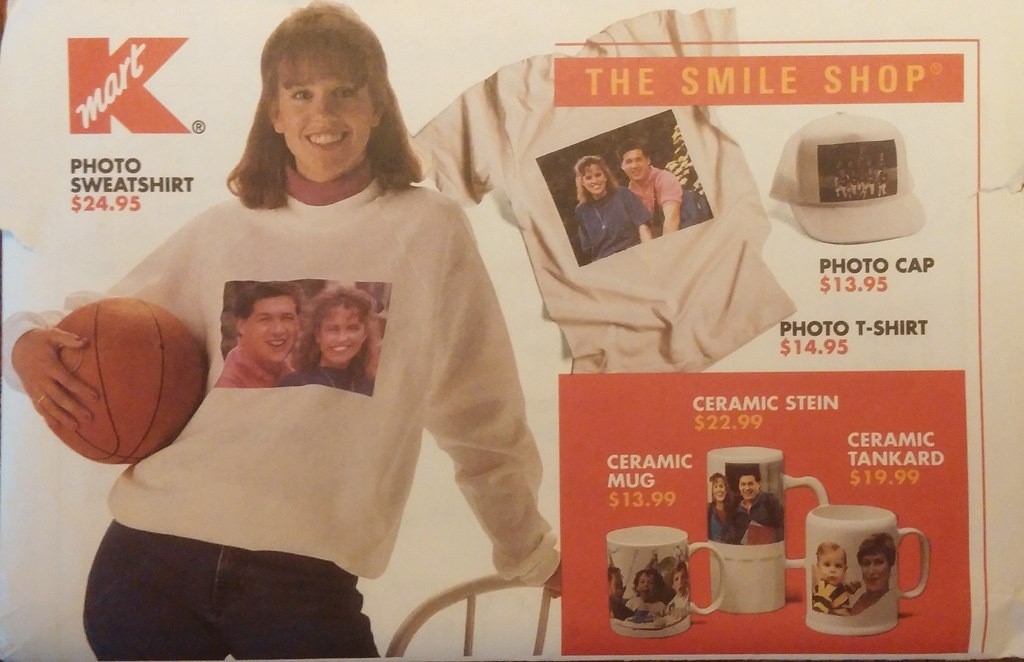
[38,394,48,403]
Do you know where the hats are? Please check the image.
[770,113,926,245]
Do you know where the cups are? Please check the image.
[708,447,827,613]
[606,526,726,638]
[806,504,931,636]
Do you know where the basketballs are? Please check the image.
[37,296,206,465]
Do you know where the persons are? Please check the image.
[217,284,382,396]
[607,549,691,627]
[572,140,695,259]
[835,153,887,200]
[811,532,897,626]
[707,473,784,544]
[1,1,560,662]
